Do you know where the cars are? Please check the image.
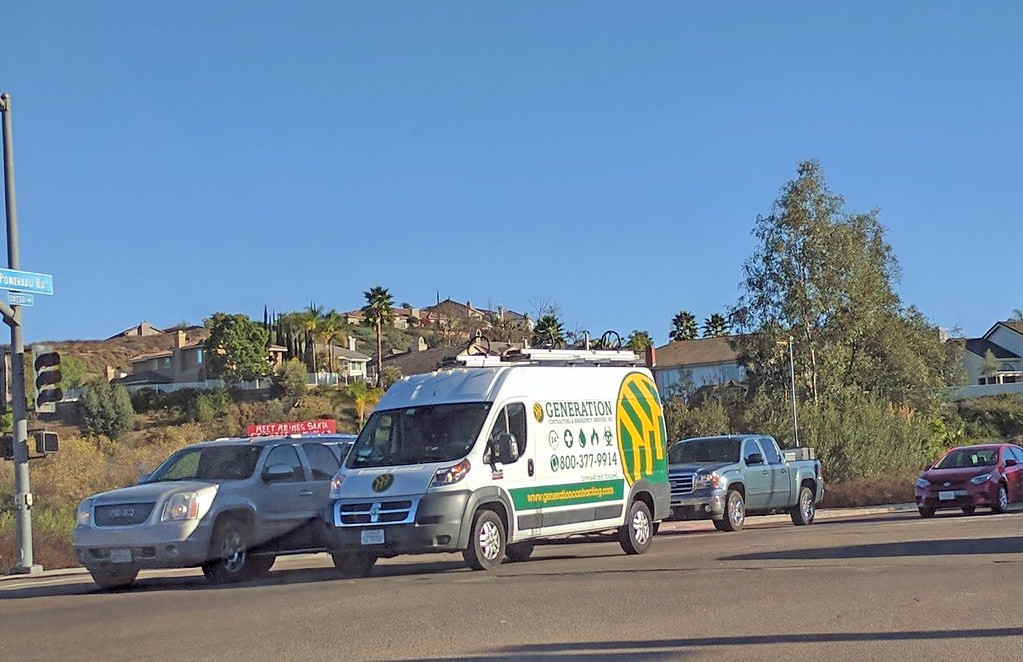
[915,444,1023,518]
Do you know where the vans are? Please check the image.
[321,349,671,575]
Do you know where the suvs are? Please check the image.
[72,434,385,589]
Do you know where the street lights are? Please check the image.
[776,336,800,448]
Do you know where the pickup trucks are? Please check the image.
[652,435,825,534]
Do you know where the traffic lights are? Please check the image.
[36,432,60,454]
[31,344,63,413]
